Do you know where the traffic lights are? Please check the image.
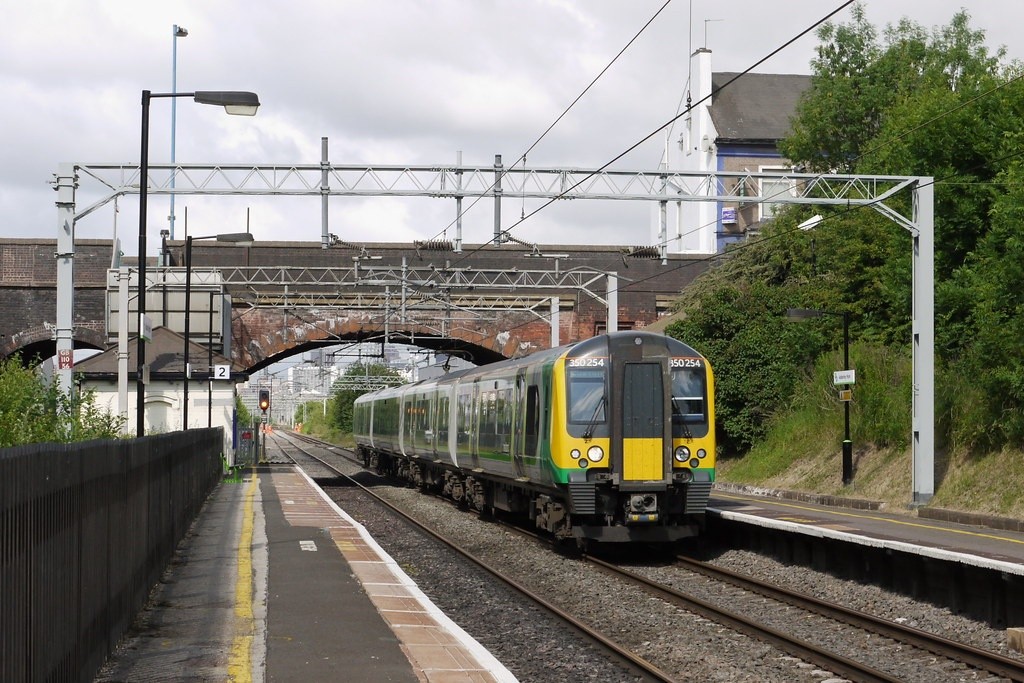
[257,390,271,412]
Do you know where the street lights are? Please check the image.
[185,230,256,432]
[167,19,188,238]
[787,305,853,484]
[126,84,262,435]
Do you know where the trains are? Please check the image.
[350,329,725,561]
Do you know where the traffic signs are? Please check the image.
[838,388,855,404]
[831,366,858,388]
